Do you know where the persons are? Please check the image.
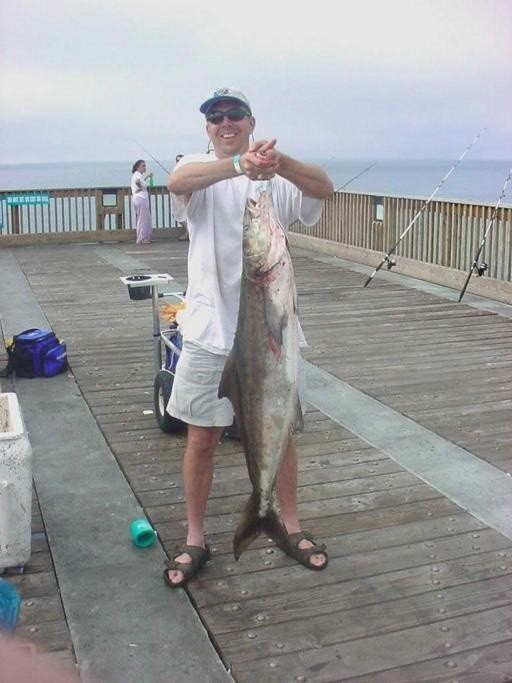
[171,154,190,241]
[163,88,334,588]
[131,159,155,245]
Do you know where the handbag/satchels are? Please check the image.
[0,328,67,377]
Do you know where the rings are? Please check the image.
[258,173,263,180]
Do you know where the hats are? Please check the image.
[199,87,252,116]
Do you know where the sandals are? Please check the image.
[163,544,211,588]
[276,523,329,570]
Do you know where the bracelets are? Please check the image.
[233,154,244,176]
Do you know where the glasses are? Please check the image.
[206,107,250,124]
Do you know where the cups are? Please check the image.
[130,519,156,548]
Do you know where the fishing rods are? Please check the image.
[459,167,512,303]
[137,140,168,175]
[364,131,479,289]
[287,161,381,227]
